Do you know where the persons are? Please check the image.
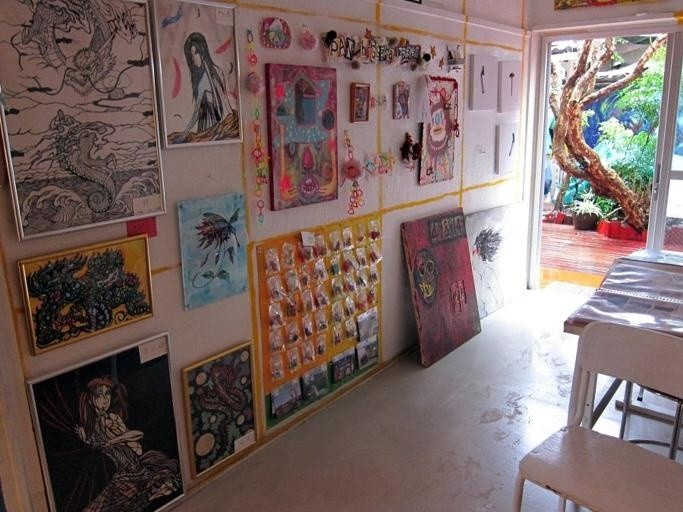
[75,377,179,511]
[176,33,234,144]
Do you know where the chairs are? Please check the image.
[512,318,683,510]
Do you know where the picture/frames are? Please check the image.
[24,330,186,512]
[17,234,154,357]
[349,81,371,125]
[1,0,169,243]
[151,1,243,150]
[180,338,257,479]
